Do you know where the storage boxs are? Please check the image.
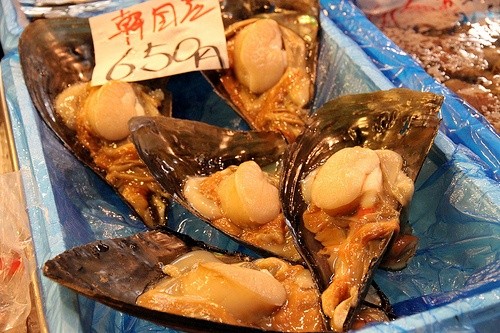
[0,0,500,333]
